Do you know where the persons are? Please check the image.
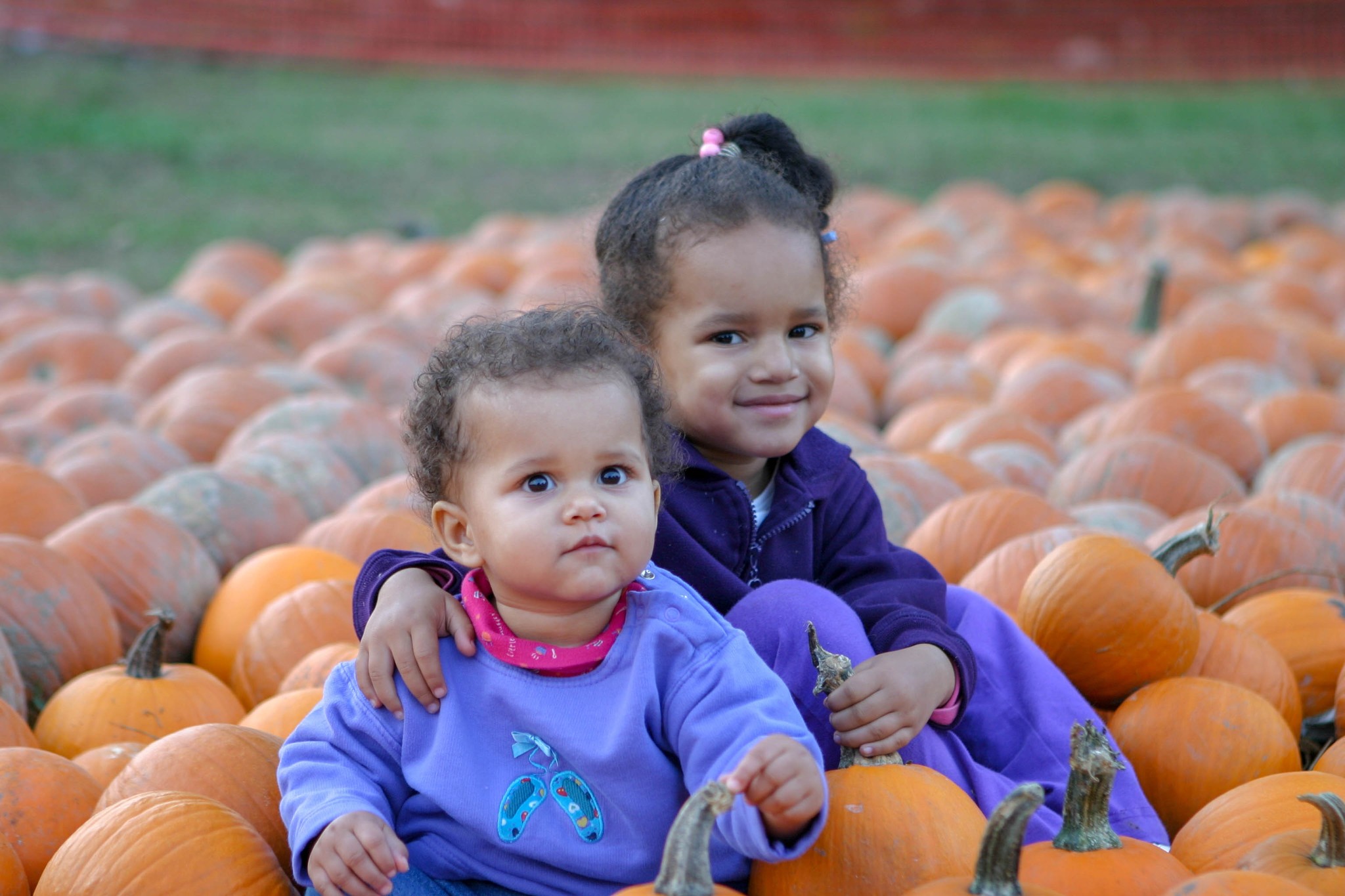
[347,111,1169,854]
[276,299,830,896]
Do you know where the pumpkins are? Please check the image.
[0,177,1345,896]
